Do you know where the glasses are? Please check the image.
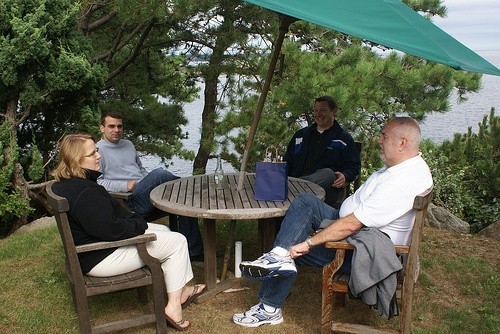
[85,147,100,157]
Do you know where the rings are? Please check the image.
[341,184,342,186]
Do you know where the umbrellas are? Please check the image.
[237,0,500,192]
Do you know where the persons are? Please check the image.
[90,110,228,262]
[231,117,434,327]
[282,95,361,210]
[51,134,207,331]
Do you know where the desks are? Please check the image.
[150,173,326,304]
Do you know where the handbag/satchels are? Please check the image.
[253,144,289,202]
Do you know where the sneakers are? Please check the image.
[232,302,284,327]
[239,252,298,282]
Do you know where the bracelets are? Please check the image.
[306,239,313,250]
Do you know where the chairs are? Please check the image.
[333,142,362,209]
[108,191,177,232]
[46,180,168,334]
[322,185,434,334]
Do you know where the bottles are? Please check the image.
[214,155,225,189]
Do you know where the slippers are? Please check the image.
[181,284,207,310]
[164,309,191,331]
